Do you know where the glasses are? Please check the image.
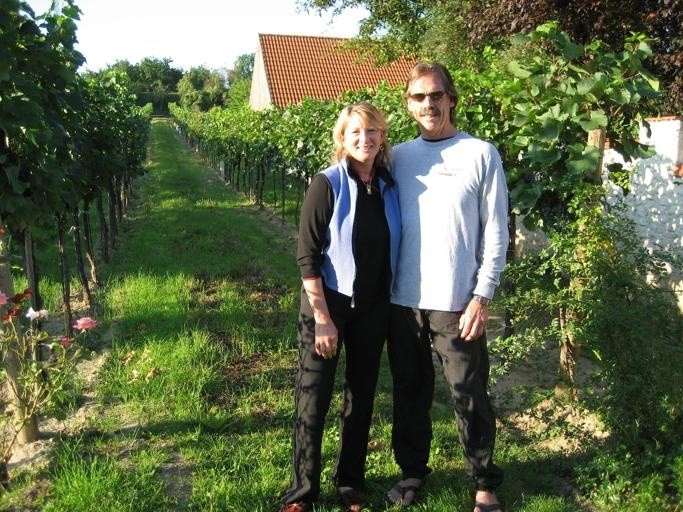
[411,90,442,101]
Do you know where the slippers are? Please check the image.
[387,474,427,507]
[474,480,502,512]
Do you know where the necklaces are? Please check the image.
[360,176,372,195]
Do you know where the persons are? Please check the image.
[278,102,403,512]
[380,61,510,512]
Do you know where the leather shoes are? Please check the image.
[279,499,307,512]
[335,484,362,511]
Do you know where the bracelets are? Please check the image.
[473,294,490,306]
